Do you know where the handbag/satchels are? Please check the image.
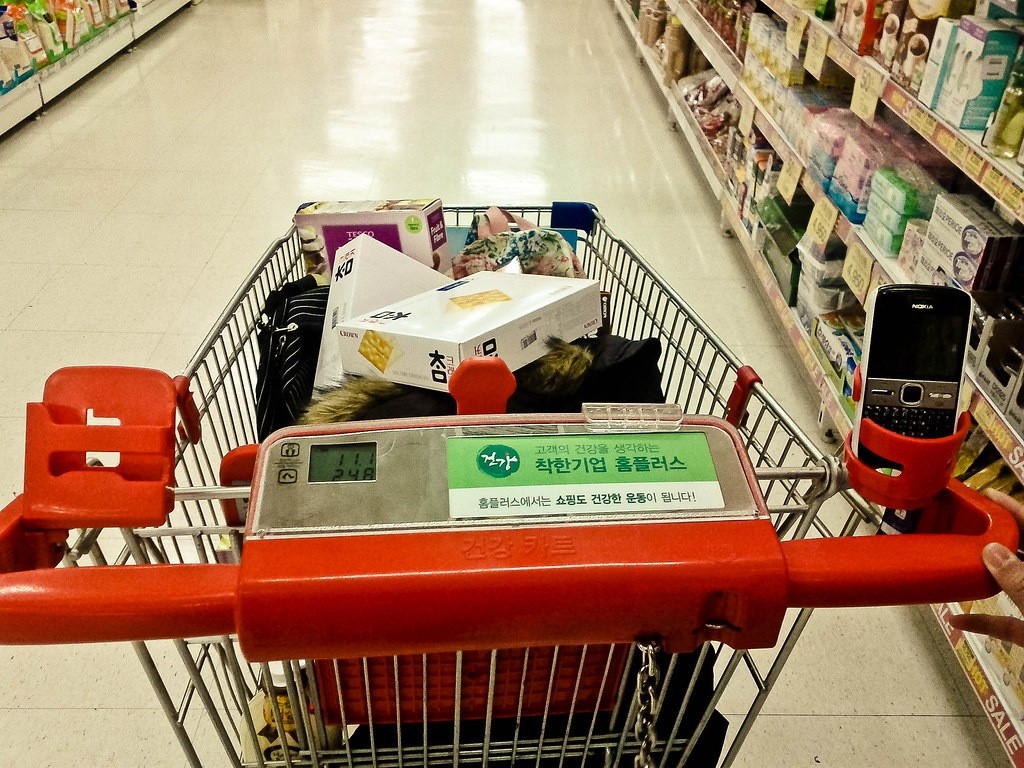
[256,273,331,443]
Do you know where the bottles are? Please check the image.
[688,38,710,76]
[664,17,688,89]
[639,1,654,44]
[987,71,1024,157]
[648,0,667,49]
[239,660,342,768]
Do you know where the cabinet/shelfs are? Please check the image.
[613,0,1024,768]
[0,0,193,136]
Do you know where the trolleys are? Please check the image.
[2,199,1022,768]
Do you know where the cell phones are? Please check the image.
[850,282,974,470]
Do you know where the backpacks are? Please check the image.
[452,207,589,282]
